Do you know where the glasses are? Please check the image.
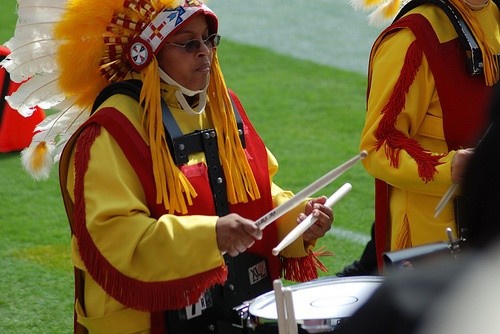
[165,34,221,54]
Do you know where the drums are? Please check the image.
[232,274,388,334]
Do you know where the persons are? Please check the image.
[58,0,333,334]
[335,0,500,334]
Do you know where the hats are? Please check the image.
[0,0,261,215]
